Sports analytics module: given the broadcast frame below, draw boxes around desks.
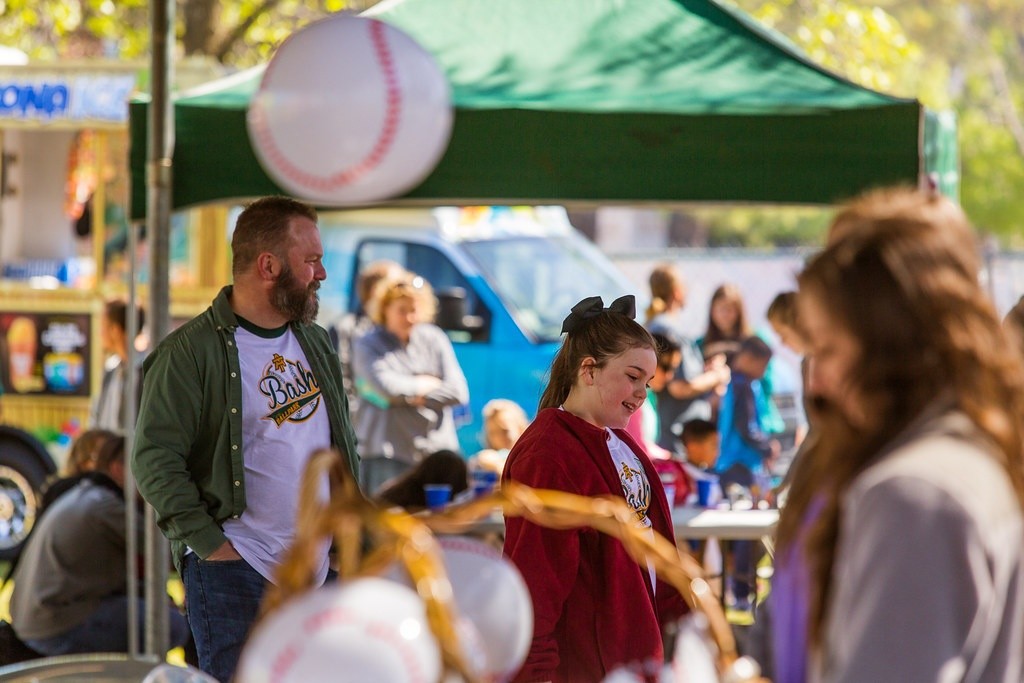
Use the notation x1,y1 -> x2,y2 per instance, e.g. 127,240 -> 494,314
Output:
670,507 -> 779,615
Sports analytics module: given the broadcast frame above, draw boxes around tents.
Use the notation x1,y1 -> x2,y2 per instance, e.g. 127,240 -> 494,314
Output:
125,0 -> 962,660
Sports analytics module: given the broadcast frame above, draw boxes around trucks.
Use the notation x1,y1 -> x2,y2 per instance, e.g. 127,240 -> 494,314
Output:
1,59 -> 650,567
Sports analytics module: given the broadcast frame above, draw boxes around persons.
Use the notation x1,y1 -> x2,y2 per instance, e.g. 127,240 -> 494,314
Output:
9,298 -> 188,656
338,256 -> 811,611
131,196 -> 359,683
499,295 -> 695,683
747,182 -> 1023,683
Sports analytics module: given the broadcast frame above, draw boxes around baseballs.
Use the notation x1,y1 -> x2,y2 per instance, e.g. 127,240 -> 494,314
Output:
245,13 -> 457,207
234,534 -> 536,683
